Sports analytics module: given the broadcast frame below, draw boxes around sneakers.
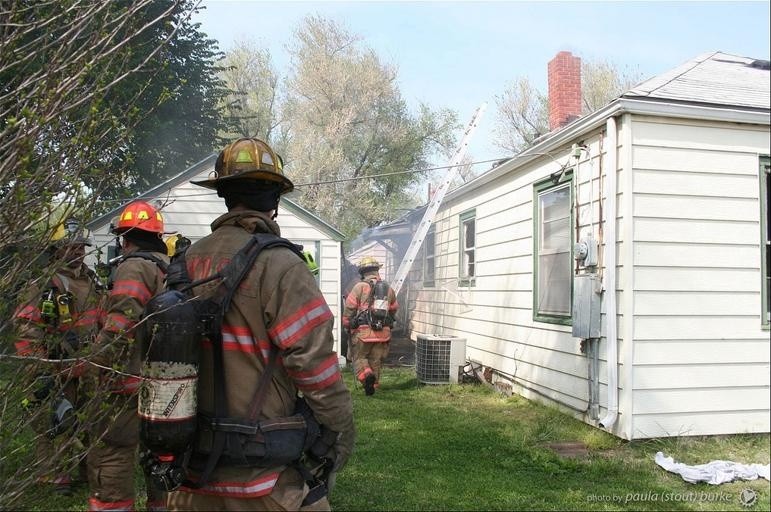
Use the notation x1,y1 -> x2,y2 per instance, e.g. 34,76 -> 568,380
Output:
364,373 -> 377,396
47,469 -> 72,500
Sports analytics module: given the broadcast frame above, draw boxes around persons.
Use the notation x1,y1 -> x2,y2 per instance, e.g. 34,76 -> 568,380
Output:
143,137 -> 356,512
343,256 -> 400,396
342,267 -> 364,367
9,198 -> 191,512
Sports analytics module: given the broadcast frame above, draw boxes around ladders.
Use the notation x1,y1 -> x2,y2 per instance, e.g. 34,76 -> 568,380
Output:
391,104 -> 486,297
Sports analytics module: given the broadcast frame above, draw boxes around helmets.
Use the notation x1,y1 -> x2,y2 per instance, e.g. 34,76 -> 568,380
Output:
112,201 -> 164,235
165,235 -> 179,258
356,257 -> 384,272
188,137 -> 295,195
48,218 -> 95,242
